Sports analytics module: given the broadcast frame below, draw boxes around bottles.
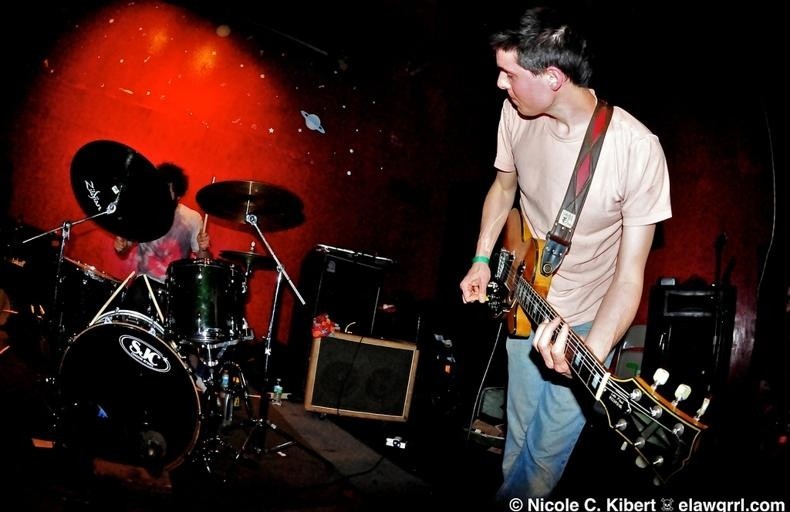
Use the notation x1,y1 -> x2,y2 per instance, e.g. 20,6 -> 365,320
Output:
221,369 -> 229,398
272,378 -> 283,404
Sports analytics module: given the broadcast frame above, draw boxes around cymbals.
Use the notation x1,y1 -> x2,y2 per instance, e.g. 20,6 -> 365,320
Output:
69,140 -> 174,243
196,179 -> 301,221
219,251 -> 279,270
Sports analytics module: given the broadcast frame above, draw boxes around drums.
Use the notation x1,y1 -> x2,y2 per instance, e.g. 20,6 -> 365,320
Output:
41,256 -> 122,330
1,223 -> 68,304
55,310 -> 204,473
162,258 -> 238,348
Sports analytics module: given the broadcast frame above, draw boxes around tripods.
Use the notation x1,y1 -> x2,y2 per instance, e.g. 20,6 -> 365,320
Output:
224,193 -> 295,469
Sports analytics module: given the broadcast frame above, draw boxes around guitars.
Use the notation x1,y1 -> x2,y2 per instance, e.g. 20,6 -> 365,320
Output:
486,207 -> 711,486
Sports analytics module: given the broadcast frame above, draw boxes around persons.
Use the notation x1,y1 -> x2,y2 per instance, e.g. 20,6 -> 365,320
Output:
112,163 -> 213,285
460,2 -> 674,512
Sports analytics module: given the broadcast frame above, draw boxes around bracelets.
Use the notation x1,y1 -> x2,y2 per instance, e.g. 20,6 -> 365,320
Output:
471,255 -> 490,265
